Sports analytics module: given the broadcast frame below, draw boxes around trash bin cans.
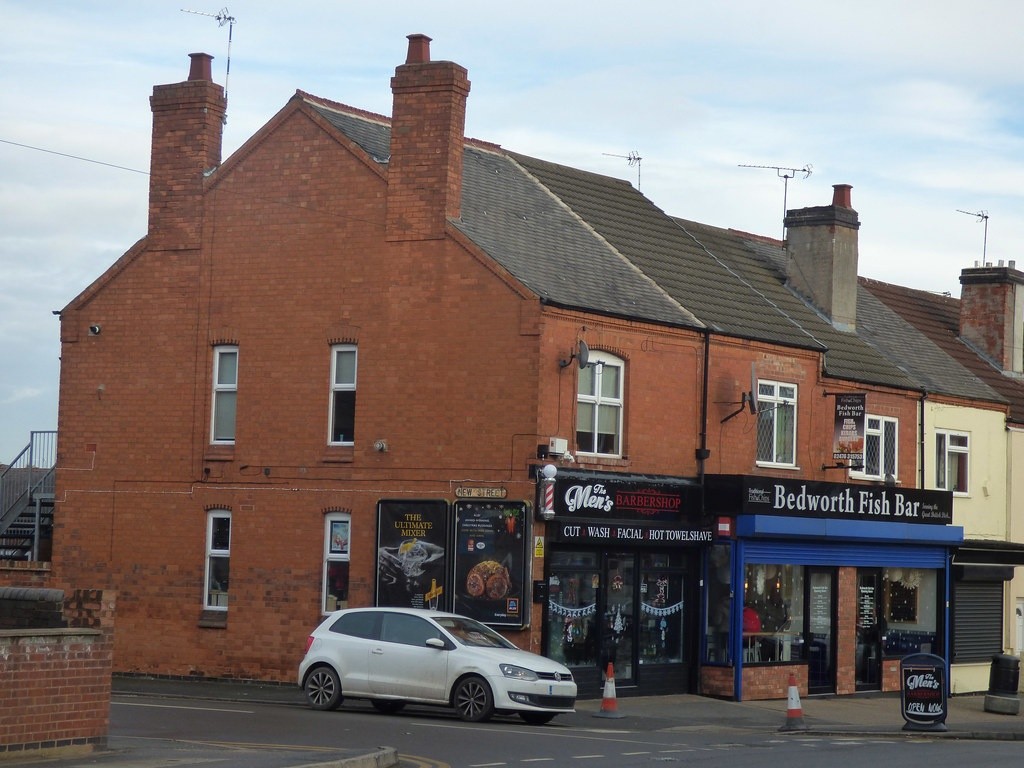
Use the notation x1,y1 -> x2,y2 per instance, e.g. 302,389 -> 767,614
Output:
988,654 -> 1021,697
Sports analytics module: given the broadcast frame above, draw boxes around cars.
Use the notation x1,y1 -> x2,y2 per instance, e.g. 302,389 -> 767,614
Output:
294,607 -> 576,728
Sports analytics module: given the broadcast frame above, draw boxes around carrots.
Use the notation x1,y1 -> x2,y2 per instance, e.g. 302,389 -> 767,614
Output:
503,508 -> 520,533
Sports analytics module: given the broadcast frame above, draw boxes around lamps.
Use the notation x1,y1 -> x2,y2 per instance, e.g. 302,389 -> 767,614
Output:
540,464 -> 557,519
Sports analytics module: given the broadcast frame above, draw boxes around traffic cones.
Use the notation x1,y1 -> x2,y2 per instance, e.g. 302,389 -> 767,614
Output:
591,663 -> 626,717
774,674 -> 818,733
